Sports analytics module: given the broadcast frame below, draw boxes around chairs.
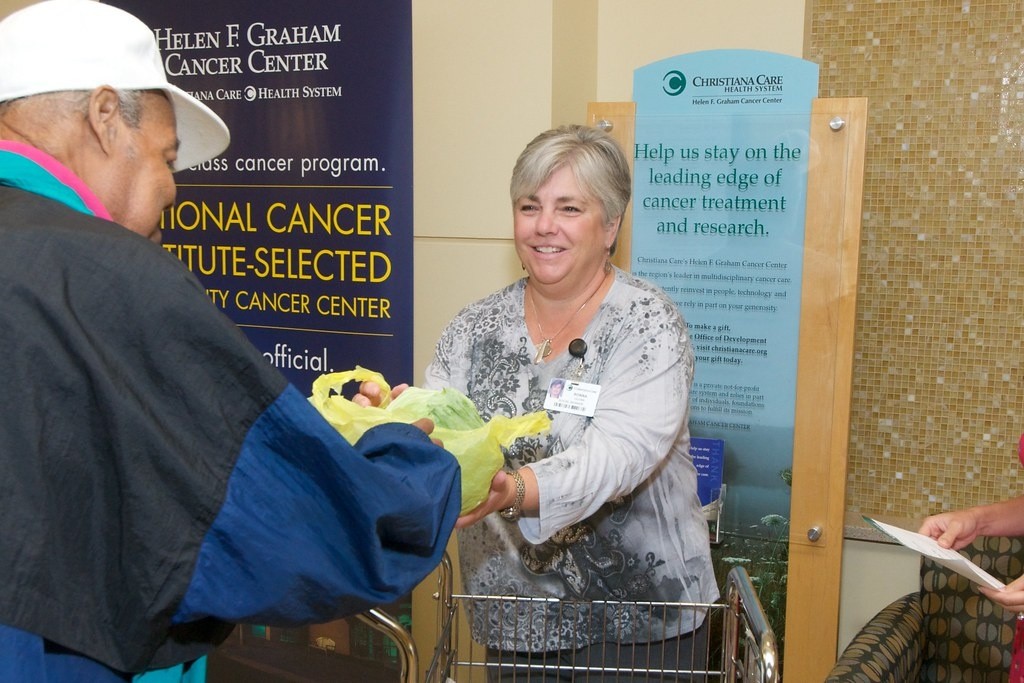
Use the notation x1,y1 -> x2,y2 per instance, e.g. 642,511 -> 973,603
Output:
823,536 -> 1024,683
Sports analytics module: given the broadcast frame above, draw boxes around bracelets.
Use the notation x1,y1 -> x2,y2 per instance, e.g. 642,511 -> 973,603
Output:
497,469 -> 526,521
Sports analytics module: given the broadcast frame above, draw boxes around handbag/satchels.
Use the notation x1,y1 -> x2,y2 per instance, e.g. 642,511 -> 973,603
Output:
305,366 -> 550,515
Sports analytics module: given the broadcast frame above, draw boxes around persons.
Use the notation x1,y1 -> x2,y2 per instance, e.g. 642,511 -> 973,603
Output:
351,125 -> 718,683
0,1 -> 464,683
917,431 -> 1023,683
549,380 -> 563,399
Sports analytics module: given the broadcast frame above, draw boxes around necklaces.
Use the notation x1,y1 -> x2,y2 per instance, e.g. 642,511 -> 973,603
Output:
528,261 -> 612,365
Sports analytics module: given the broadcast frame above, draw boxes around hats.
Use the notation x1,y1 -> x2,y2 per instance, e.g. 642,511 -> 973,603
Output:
1,0 -> 230,173
